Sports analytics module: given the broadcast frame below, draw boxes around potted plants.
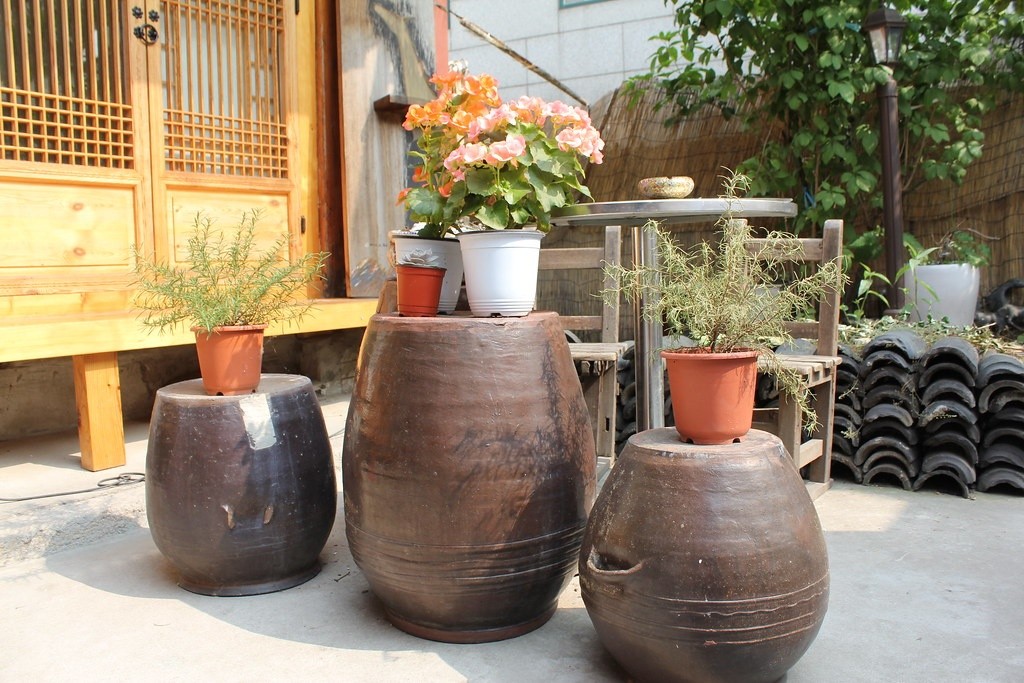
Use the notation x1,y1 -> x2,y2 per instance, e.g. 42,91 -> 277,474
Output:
904,220 -> 1002,329
122,208 -> 332,397
588,165 -> 853,444
394,248 -> 447,317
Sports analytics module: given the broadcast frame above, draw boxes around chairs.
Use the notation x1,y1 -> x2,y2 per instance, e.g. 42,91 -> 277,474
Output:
729,218 -> 844,481
537,225 -> 624,457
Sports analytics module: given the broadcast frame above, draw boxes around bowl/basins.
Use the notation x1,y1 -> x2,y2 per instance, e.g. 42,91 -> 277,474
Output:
638,175 -> 695,199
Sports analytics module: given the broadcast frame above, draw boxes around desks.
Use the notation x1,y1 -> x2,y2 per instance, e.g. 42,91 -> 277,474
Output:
541,200 -> 798,432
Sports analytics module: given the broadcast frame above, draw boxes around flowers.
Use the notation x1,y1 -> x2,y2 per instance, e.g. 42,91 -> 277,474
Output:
394,61 -> 605,238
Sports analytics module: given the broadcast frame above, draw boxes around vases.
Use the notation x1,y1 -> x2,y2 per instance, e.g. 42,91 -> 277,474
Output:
456,230 -> 544,316
392,236 -> 463,314
